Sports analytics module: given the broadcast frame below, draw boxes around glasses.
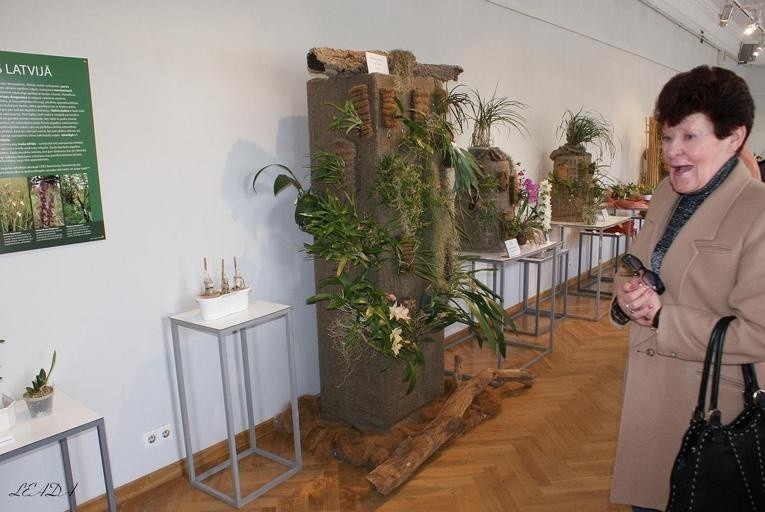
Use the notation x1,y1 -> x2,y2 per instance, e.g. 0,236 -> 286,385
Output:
618,254 -> 665,295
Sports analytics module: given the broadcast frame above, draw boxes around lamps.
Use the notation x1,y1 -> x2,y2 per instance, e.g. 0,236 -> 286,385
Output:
719,2 -> 734,28
752,33 -> 765,56
743,9 -> 761,36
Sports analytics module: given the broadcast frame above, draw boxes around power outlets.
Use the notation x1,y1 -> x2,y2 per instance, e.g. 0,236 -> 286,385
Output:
143,423 -> 174,449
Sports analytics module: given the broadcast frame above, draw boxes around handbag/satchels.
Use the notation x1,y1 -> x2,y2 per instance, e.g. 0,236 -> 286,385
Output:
663,314 -> 765,511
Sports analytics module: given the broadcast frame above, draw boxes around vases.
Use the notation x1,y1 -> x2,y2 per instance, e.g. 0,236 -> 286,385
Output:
516,233 -> 526,245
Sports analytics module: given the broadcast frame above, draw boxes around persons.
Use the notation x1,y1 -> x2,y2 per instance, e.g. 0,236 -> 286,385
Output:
609,65 -> 765,512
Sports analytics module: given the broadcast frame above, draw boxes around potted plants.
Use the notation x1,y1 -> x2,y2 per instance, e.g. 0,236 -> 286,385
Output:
549,107 -> 617,221
572,180 -> 605,224
458,81 -> 530,253
611,182 -> 656,201
0,338 -> 18,432
23,349 -> 57,417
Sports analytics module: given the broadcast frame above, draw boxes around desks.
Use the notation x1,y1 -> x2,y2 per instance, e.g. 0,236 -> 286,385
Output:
491,248 -> 568,337
445,241 -> 563,379
169,302 -> 303,509
577,215 -> 645,296
610,197 -> 652,219
526,215 -> 633,323
0,394 -> 116,511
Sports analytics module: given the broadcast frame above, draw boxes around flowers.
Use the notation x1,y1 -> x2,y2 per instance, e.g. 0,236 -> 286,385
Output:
509,179 -> 552,245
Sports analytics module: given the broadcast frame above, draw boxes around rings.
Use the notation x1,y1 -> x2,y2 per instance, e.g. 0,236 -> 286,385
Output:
625,304 -> 636,313
